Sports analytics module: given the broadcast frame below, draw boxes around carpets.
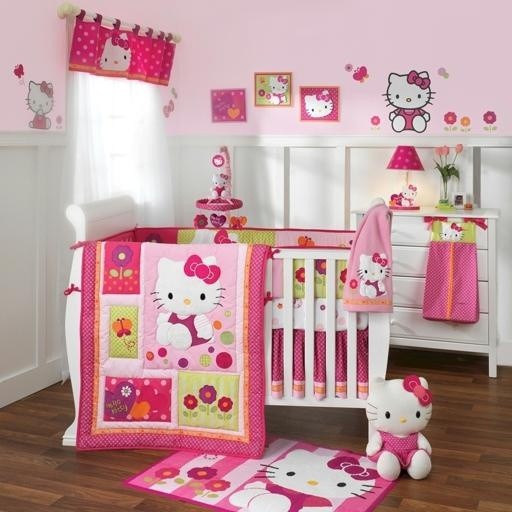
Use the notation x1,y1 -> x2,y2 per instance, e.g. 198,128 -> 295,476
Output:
122,431 -> 400,512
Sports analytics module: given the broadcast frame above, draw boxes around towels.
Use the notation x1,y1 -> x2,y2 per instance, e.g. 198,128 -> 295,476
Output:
342,204 -> 393,313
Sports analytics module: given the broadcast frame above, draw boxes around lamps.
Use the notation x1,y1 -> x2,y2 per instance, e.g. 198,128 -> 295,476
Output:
385,144 -> 426,210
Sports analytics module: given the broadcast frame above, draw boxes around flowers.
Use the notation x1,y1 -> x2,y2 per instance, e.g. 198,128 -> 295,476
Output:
432,144 -> 464,177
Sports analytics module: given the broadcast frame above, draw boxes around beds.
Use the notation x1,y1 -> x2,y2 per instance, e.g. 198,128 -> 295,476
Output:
62,194 -> 390,447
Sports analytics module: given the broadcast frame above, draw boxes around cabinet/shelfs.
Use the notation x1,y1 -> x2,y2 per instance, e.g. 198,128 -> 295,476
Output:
349,207 -> 499,378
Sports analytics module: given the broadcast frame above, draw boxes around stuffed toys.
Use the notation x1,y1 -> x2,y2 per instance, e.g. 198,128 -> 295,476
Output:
210,174 -> 230,198
365,375 -> 433,481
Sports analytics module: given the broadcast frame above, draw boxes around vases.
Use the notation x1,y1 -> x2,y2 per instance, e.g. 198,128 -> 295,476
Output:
439,176 -> 448,204
